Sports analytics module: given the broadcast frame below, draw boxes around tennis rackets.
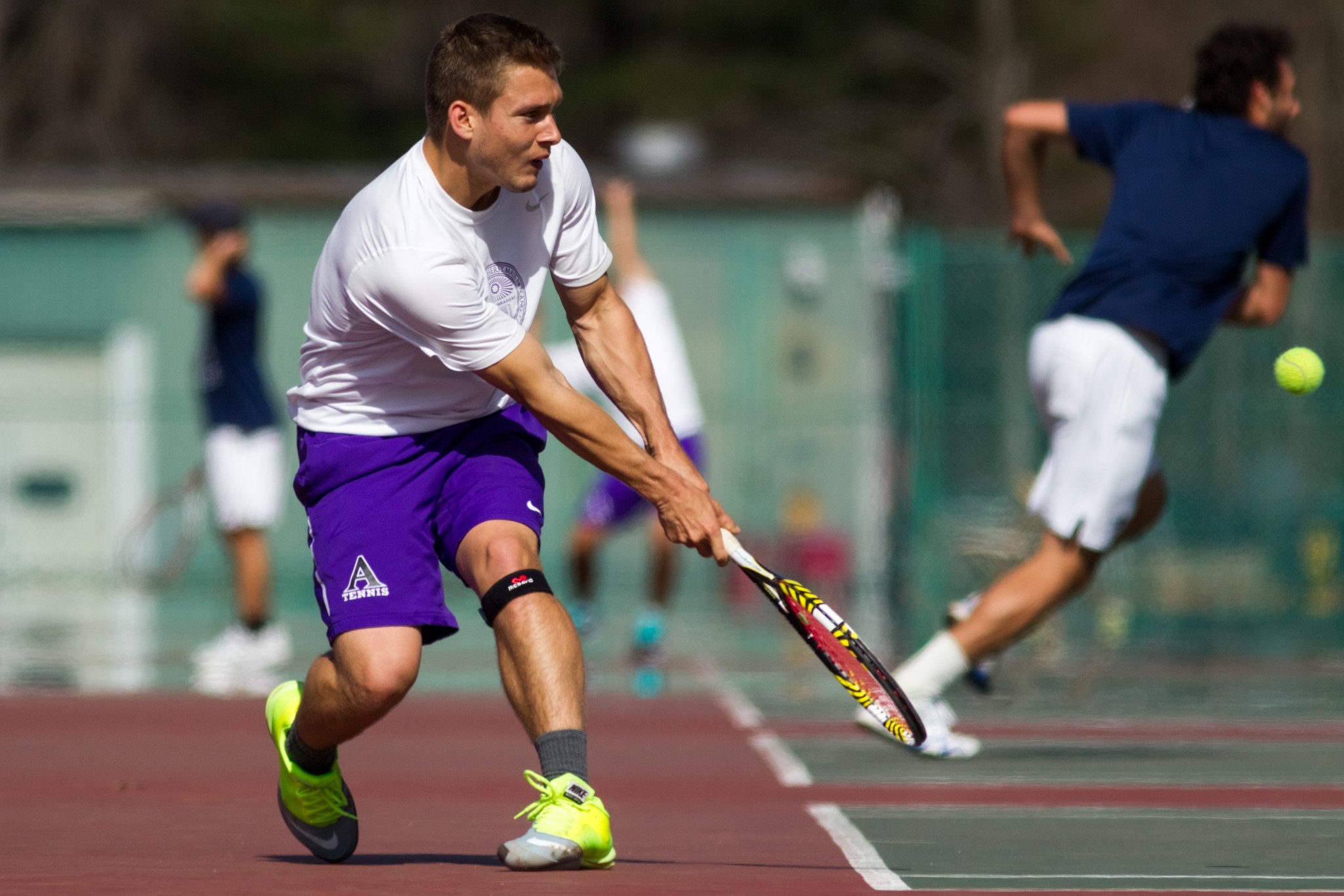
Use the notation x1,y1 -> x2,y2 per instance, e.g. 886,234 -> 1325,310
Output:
717,527 -> 928,747
118,467 -> 206,593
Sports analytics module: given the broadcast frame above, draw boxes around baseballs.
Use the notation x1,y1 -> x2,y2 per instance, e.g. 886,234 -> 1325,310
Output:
1274,347 -> 1326,394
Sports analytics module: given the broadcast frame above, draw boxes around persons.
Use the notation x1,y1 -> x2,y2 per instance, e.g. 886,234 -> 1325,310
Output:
188,201 -> 295,702
266,12 -> 739,871
534,174 -> 711,672
851,21 -> 1310,763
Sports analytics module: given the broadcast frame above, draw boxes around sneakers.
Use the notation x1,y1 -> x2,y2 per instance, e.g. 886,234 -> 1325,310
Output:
853,693 -> 981,760
946,589 -> 999,696
265,682 -> 359,860
498,772 -> 616,870
